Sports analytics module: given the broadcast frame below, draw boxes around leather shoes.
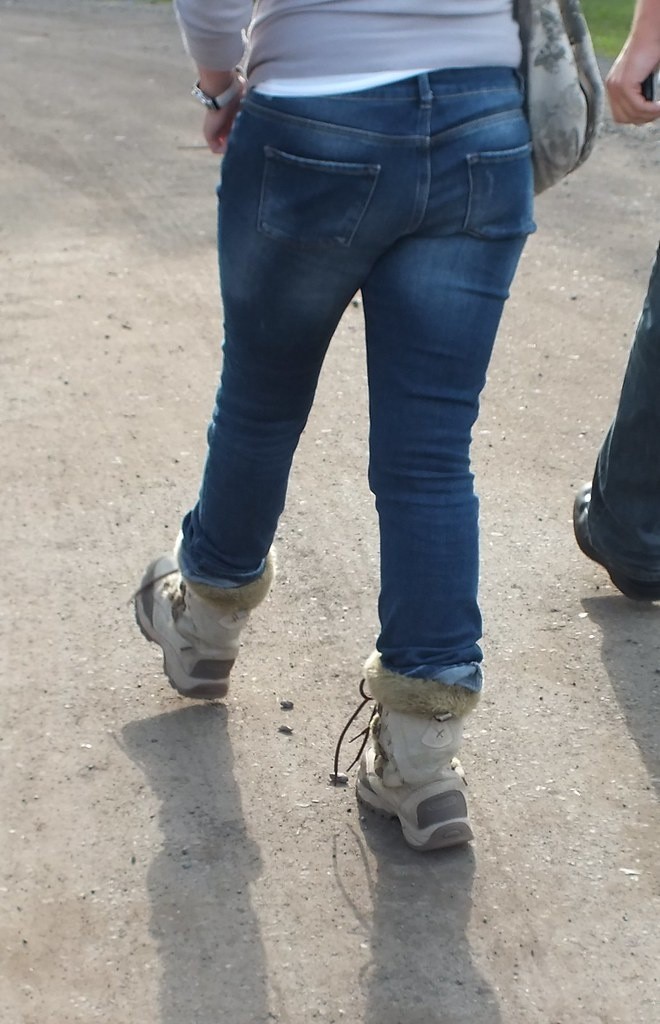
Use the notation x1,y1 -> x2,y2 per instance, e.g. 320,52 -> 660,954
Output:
572,479 -> 660,602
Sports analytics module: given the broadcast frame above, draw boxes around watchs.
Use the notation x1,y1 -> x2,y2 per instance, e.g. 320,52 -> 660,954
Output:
192,64 -> 244,110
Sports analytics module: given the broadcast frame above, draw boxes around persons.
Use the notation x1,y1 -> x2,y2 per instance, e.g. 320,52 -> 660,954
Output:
573,1 -> 659,605
126,0 -> 534,850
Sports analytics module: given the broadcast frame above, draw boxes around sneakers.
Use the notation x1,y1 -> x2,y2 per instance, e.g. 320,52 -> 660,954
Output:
354,646 -> 481,853
134,526 -> 277,700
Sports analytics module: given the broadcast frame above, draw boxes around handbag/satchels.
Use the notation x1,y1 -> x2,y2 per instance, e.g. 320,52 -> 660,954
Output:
512,0 -> 607,196
